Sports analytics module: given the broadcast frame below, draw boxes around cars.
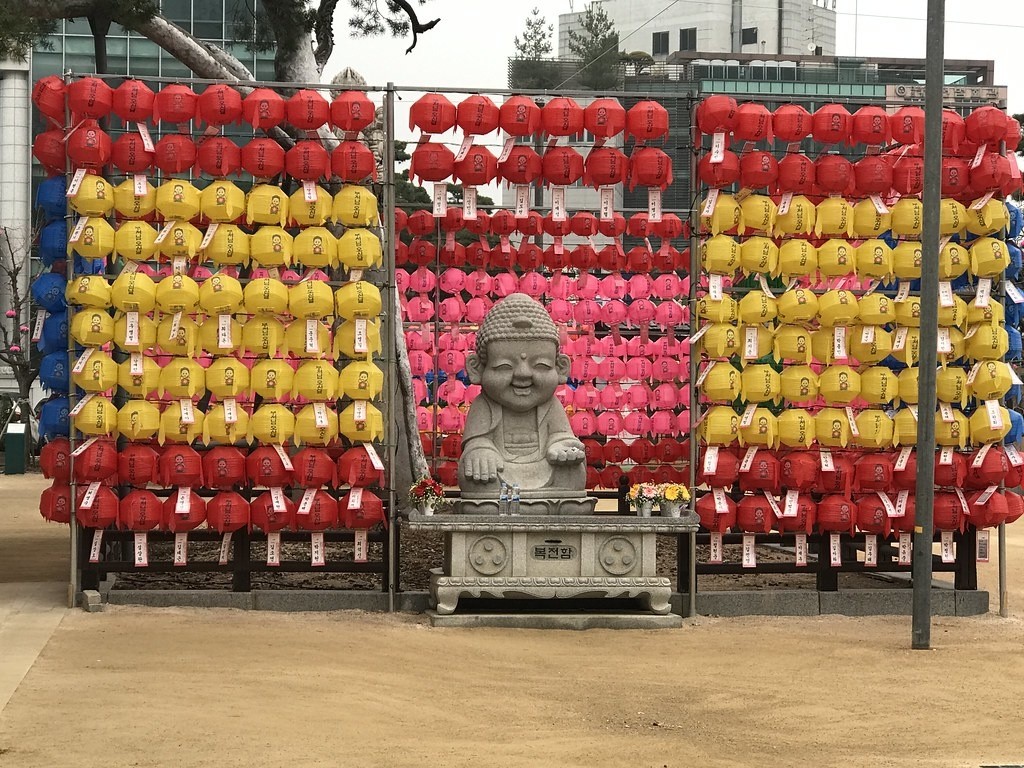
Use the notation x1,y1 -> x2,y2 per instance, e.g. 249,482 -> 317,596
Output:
0,411 -> 49,457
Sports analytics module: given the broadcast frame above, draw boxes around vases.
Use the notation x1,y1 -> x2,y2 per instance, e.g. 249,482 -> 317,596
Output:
418,501 -> 435,516
660,500 -> 681,517
636,502 -> 652,517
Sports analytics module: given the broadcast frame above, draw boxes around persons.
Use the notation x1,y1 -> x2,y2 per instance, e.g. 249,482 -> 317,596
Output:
457,292 -> 589,498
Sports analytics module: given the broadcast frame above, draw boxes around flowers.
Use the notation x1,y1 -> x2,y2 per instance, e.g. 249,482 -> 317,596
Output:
410,475 -> 444,507
624,482 -> 659,507
658,480 -> 691,509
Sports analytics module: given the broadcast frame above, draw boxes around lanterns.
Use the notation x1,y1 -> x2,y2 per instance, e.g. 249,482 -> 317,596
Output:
391,93 -> 692,486
696,96 -> 1024,536
33,75 -> 386,532
